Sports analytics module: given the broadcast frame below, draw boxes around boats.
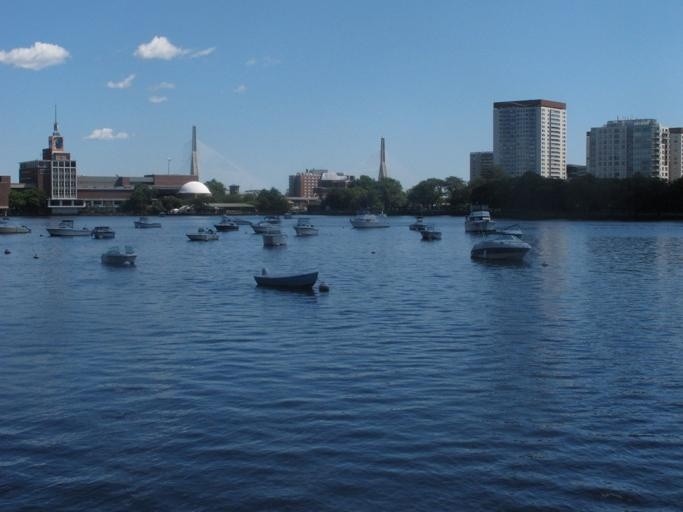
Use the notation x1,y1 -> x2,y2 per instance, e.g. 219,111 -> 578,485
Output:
459,203 -> 531,264
253,266 -> 320,290
